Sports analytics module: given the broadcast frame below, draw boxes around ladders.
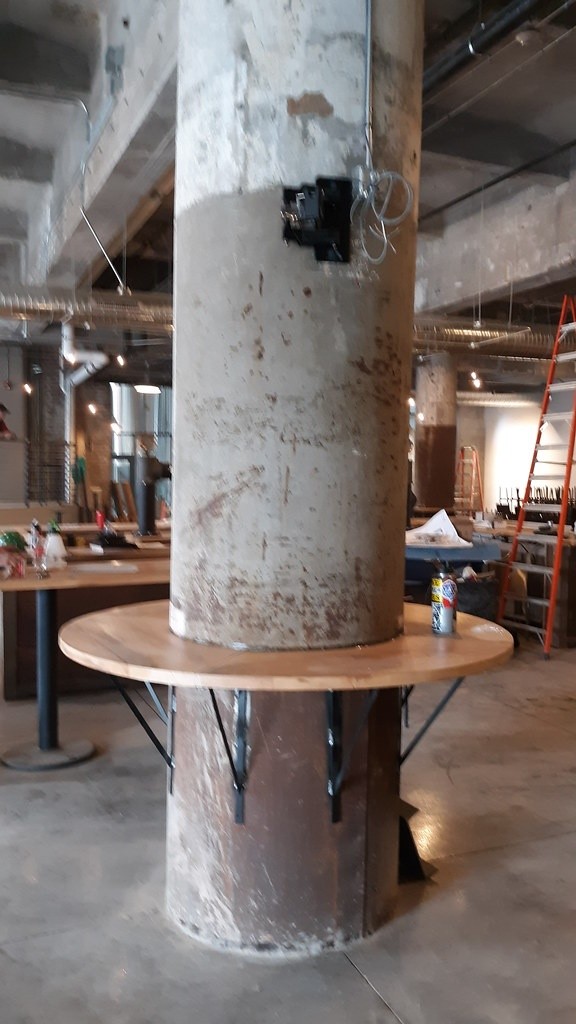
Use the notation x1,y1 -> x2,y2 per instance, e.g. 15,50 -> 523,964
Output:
454,445 -> 484,519
497,294 -> 576,661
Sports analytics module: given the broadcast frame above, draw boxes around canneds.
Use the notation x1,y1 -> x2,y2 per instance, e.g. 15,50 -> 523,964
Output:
431,572 -> 456,635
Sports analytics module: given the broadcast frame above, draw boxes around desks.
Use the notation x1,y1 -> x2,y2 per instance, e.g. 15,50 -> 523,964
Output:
0,513 -> 171,774
58,599 -> 513,825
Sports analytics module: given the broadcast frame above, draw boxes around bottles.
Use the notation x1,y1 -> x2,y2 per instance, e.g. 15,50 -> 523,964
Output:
431,567 -> 458,637
30,519 -> 50,580
